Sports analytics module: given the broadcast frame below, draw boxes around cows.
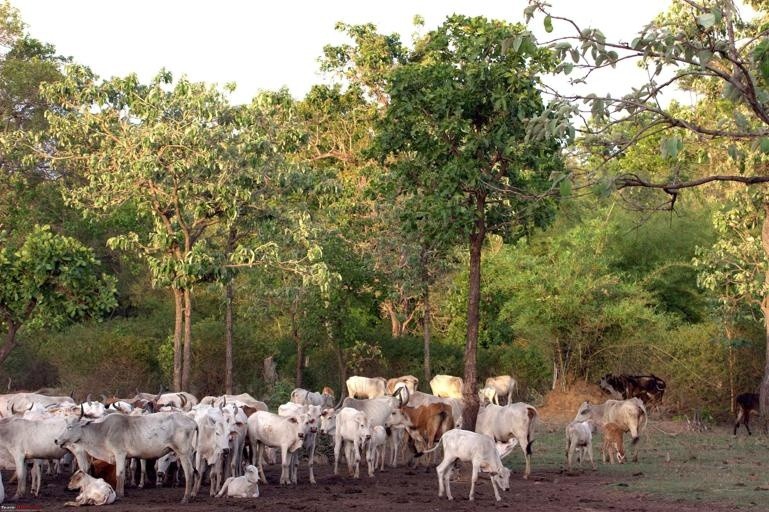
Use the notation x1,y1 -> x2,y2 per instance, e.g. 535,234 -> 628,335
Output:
0,375 -> 538,506
566,397 -> 648,473
599,373 -> 664,413
734,393 -> 760,436
